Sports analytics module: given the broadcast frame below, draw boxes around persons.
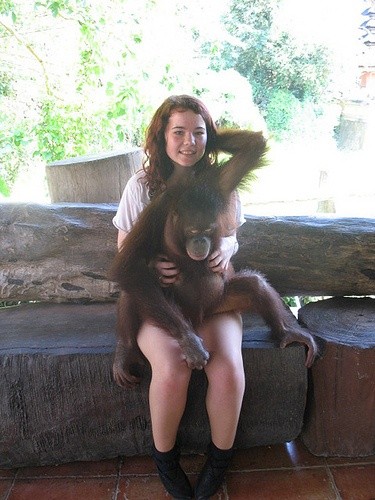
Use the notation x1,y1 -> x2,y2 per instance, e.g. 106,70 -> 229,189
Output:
113,94 -> 247,499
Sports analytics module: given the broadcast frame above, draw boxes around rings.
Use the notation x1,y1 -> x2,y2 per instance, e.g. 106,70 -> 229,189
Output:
158,274 -> 164,280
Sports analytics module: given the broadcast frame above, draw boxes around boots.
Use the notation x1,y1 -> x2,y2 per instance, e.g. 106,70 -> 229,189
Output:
152,439 -> 194,500
194,437 -> 236,500
